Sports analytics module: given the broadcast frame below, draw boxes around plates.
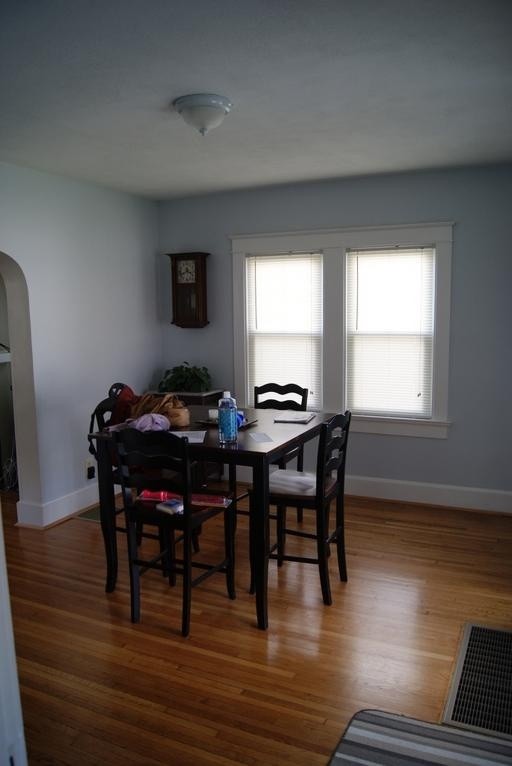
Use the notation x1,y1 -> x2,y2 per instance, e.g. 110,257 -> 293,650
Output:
194,416 -> 258,429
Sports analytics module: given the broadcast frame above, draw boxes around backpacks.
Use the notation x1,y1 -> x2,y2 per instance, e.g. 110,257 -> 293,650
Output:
93,383 -> 142,466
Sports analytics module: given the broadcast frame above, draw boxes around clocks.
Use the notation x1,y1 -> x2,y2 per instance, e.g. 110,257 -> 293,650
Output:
159,251 -> 210,329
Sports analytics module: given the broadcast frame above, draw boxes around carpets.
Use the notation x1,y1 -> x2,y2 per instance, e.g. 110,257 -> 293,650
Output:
330,705 -> 512,766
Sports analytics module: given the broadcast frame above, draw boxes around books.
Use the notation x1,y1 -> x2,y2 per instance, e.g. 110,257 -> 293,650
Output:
170,430 -> 209,443
273,408 -> 316,423
154,496 -> 184,516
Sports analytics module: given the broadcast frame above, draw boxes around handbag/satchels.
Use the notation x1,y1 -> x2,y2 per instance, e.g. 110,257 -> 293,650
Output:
125,392 -> 189,433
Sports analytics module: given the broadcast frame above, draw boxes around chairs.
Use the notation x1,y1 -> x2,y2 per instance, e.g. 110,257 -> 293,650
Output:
88,382 -> 352,638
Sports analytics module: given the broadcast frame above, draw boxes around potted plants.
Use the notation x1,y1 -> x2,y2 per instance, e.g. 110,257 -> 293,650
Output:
159,361 -> 211,393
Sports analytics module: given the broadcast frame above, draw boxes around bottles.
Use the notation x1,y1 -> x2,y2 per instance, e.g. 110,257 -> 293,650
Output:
218,391 -> 238,444
